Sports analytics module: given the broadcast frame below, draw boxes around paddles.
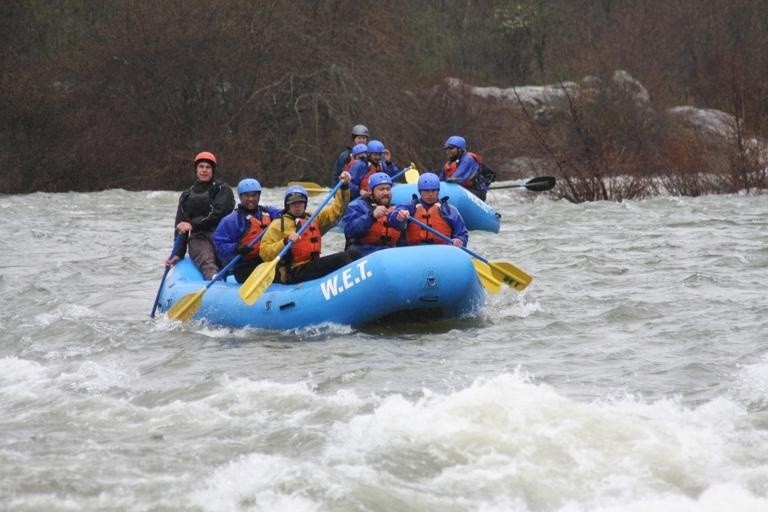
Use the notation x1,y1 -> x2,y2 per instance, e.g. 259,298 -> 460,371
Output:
239,172 -> 346,305
168,228 -> 270,323
288,182 -> 333,197
471,257 -> 505,293
478,177 -> 556,191
408,216 -> 532,291
404,168 -> 420,183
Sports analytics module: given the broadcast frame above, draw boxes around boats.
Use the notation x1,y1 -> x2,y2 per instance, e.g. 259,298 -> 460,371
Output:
157,239 -> 489,336
327,171 -> 502,235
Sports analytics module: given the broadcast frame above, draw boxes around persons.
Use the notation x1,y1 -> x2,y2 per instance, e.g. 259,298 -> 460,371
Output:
332,123 -> 488,266
165,152 -> 236,284
258,170 -> 363,284
210,178 -> 283,284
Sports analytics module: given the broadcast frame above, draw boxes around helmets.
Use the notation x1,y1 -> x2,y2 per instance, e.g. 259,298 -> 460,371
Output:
352,124 -> 369,137
418,172 -> 440,191
237,177 -> 262,194
368,172 -> 393,189
366,140 -> 385,153
284,184 -> 308,210
352,143 -> 367,157
194,151 -> 217,166
445,135 -> 466,150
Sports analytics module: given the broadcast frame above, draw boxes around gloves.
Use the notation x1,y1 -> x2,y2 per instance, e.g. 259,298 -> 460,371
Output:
237,244 -> 251,256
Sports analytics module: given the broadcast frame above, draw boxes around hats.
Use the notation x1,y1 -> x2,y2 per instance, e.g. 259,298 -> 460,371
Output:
286,192 -> 307,205
